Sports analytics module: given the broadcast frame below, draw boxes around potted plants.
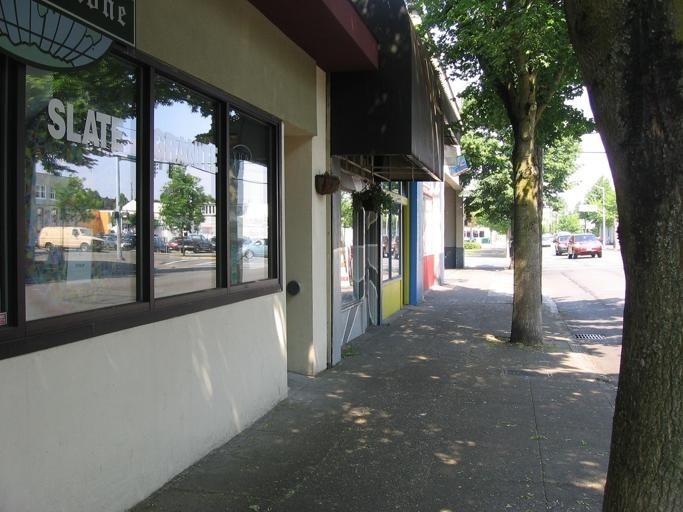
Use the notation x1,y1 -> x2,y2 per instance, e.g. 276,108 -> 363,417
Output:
351,181 -> 398,219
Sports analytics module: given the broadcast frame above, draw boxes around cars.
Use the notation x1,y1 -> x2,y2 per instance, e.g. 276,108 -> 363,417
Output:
38,226 -> 268,259
542,234 -> 603,258
